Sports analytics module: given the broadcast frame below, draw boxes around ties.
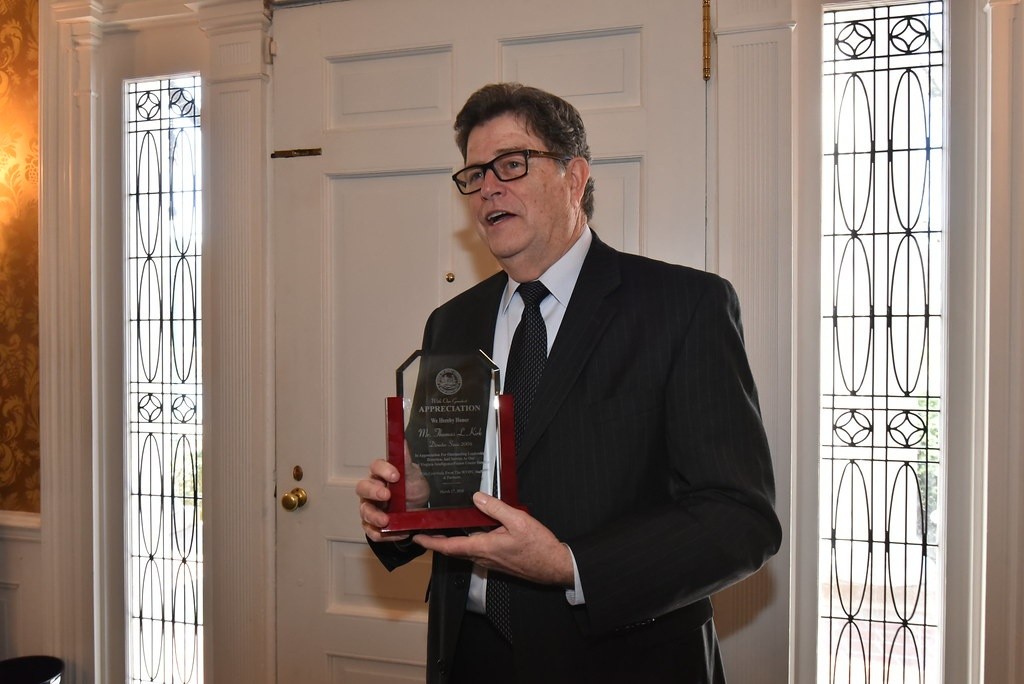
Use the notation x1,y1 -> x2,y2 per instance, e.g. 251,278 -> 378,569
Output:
485,281 -> 551,645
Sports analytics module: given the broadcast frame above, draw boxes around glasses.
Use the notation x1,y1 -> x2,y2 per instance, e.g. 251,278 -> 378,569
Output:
451,148 -> 573,195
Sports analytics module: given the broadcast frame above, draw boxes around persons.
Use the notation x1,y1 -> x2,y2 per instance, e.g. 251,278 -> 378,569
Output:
354,84 -> 784,684
